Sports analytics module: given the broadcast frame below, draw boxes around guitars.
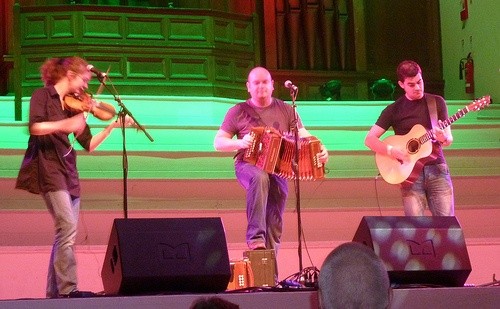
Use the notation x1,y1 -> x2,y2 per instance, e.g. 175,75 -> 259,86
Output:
375,93 -> 492,188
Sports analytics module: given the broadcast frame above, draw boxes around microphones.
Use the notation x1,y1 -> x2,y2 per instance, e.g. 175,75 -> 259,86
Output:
87,65 -> 107,77
285,80 -> 297,91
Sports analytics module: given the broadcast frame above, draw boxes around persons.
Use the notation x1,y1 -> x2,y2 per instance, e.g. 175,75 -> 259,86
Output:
14,55 -> 132,298
215,66 -> 329,290
365,60 -> 454,216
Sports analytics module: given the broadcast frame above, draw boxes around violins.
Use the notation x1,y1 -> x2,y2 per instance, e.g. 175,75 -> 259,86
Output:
62,91 -> 146,132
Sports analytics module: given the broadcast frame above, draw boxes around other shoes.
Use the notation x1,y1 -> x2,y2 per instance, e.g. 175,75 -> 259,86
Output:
249,242 -> 266,250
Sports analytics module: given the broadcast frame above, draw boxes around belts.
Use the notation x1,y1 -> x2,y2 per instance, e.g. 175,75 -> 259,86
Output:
423,158 -> 445,166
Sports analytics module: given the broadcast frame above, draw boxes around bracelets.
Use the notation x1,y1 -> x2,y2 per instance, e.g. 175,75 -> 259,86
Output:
387,145 -> 392,155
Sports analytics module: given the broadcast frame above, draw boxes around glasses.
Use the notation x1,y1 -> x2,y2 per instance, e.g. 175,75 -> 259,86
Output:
75,73 -> 88,84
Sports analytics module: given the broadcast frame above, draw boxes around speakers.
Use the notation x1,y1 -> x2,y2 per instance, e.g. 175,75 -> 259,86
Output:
101,217 -> 231,296
352,216 -> 472,287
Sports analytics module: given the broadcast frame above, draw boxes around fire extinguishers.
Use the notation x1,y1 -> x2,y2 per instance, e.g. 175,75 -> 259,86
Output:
457,51 -> 475,94
